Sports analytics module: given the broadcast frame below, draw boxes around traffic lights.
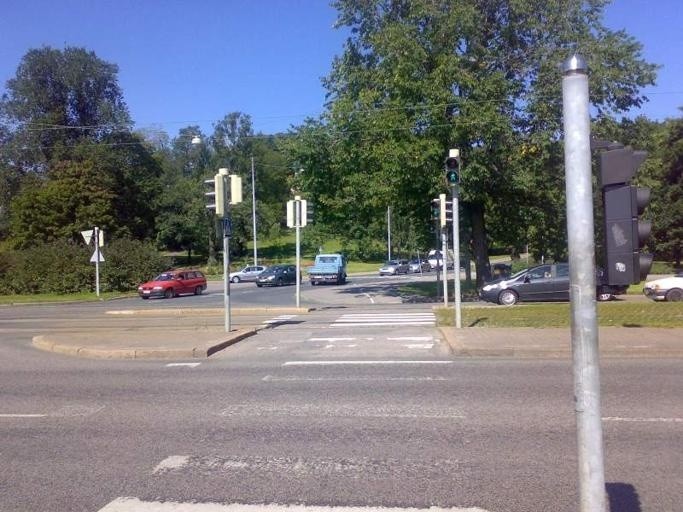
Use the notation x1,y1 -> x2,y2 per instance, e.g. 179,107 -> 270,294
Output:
203,174 -> 226,218
445,156 -> 461,189
600,144 -> 655,286
428,194 -> 454,226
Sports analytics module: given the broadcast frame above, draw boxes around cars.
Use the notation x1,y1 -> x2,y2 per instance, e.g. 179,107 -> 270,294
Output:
378,259 -> 410,276
643,269 -> 683,303
228,265 -> 268,283
138,270 -> 207,299
255,264 -> 302,288
477,261 -> 629,306
409,248 -> 456,273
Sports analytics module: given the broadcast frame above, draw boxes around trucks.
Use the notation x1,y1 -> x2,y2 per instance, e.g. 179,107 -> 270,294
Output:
306,254 -> 347,285
